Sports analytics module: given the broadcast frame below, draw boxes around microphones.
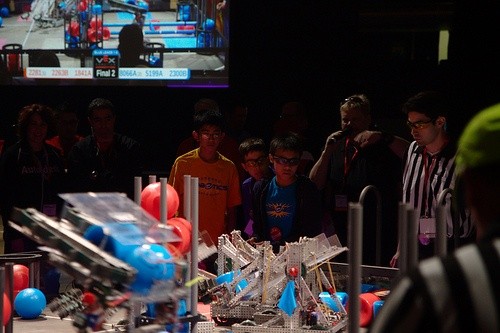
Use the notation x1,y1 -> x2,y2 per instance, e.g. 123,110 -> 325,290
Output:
327,126 -> 352,145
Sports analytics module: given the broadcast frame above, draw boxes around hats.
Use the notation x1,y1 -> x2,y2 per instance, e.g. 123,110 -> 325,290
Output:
453,103 -> 500,175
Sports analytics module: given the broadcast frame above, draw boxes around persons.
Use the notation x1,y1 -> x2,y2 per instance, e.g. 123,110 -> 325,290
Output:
367,104 -> 500,333
175,94 -> 242,166
46,105 -> 86,158
248,132 -> 336,252
220,102 -> 250,146
0,103 -> 68,253
60,98 -> 141,201
274,99 -> 328,179
309,93 -> 411,264
167,109 -> 242,247
388,92 -> 475,272
387,136 -> 412,175
237,137 -> 273,237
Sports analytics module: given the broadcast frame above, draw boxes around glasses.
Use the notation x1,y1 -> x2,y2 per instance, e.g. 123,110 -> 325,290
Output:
341,96 -> 364,106
273,156 -> 300,165
244,154 -> 269,167
407,121 -> 432,129
